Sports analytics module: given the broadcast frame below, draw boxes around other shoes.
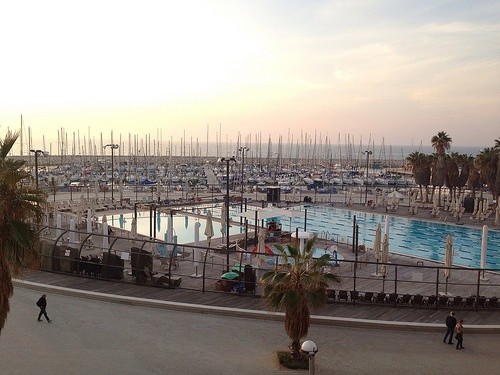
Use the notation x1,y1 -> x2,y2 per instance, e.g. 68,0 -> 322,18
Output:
461,347 -> 464,349
48,319 -> 52,322
456,347 -> 459,349
38,319 -> 42,321
443,340 -> 446,343
449,342 -> 453,344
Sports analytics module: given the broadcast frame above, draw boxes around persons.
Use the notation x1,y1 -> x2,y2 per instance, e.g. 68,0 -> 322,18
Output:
108,225 -> 114,244
455,320 -> 466,350
36,293 -> 52,323
443,311 -> 457,345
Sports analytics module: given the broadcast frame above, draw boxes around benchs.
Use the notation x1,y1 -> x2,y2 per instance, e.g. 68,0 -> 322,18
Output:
153,274 -> 181,286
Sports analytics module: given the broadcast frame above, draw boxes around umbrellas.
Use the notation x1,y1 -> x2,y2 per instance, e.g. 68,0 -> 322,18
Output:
372,215 -> 389,278
76,204 -> 82,243
220,203 -> 227,244
257,229 -> 266,269
384,187 -> 500,225
131,218 -> 137,243
444,234 -> 454,294
204,210 -> 214,253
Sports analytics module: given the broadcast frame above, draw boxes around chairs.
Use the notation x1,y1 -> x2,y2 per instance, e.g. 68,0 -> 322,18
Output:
350,290 -> 500,307
326,289 -> 336,300
338,290 -> 348,301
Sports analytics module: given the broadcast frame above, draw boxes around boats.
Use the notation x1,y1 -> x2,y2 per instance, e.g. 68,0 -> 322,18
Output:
18,112 -> 425,188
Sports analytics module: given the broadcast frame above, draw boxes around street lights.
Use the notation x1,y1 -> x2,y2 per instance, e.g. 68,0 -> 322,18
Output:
103,143 -> 119,202
217,156 -> 241,270
31,149 -> 48,236
238,146 -> 250,226
362,150 -> 373,204
